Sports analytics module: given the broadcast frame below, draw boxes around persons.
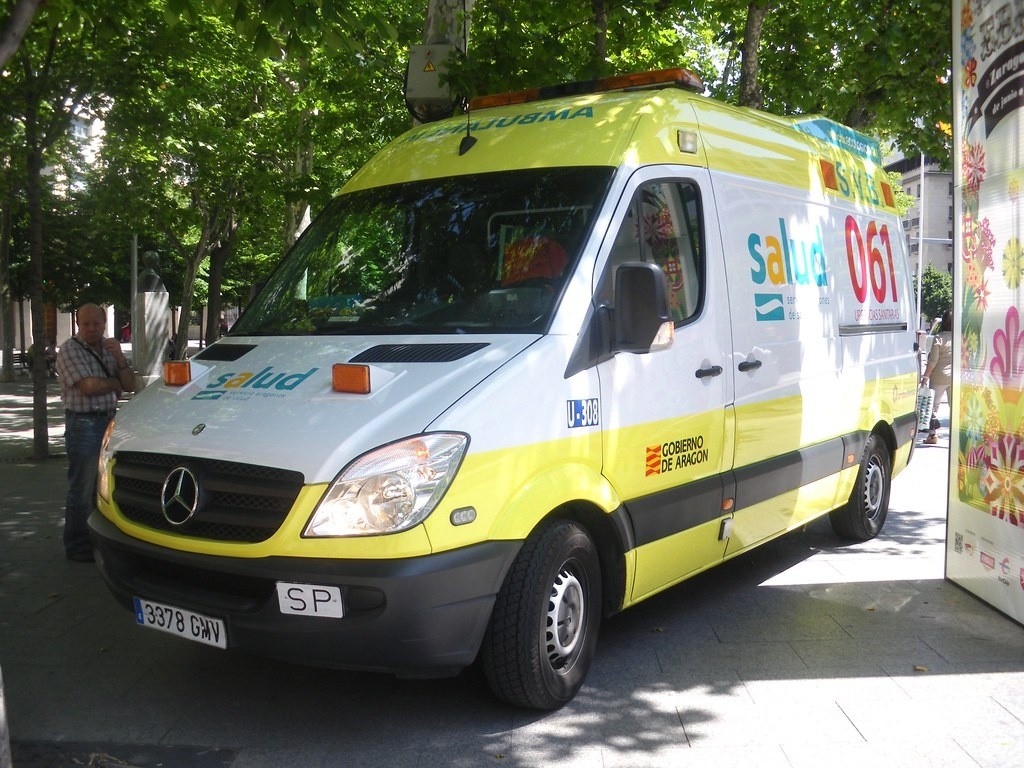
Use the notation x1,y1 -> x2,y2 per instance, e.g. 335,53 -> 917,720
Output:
118,309 -> 131,342
920,312 -> 953,444
53,303 -> 135,562
500,239 -> 653,351
219,319 -> 229,337
169,334 -> 177,359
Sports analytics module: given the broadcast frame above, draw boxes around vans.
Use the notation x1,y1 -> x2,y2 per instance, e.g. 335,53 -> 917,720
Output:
85,72 -> 926,715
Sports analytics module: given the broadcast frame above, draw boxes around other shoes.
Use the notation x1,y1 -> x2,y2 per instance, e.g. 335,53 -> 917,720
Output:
65,549 -> 94,563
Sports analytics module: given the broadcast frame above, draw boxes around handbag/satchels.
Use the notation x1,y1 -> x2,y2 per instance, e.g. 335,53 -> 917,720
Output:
917,381 -> 935,430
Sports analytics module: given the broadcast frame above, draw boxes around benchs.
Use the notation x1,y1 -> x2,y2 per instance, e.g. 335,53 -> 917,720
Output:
13,353 -> 28,363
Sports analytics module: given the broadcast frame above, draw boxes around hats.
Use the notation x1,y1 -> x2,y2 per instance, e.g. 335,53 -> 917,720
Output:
500,238 -> 568,286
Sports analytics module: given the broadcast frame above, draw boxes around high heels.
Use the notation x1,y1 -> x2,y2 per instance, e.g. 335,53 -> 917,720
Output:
923,434 -> 938,444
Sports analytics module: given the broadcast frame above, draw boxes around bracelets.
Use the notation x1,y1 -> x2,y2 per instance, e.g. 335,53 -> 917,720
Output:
922,375 -> 928,378
118,357 -> 132,369
